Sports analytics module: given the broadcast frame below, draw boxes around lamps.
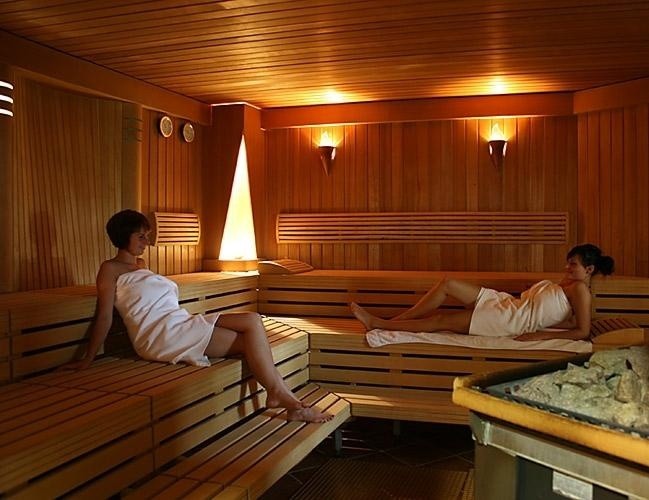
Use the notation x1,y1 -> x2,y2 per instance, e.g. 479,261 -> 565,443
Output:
314,129 -> 336,176
485,121 -> 509,168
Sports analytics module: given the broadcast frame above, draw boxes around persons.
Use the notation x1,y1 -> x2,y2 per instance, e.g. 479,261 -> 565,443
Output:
350,242 -> 615,342
58,208 -> 335,423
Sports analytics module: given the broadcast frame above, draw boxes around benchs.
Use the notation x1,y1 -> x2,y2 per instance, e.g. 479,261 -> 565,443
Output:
0,263 -> 649,500
